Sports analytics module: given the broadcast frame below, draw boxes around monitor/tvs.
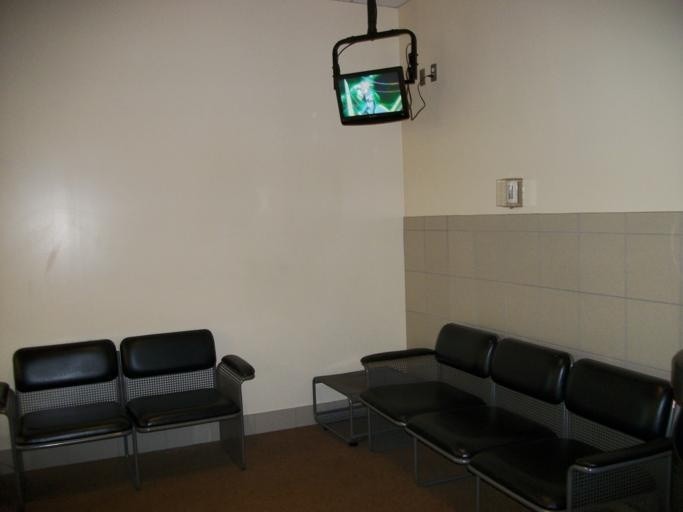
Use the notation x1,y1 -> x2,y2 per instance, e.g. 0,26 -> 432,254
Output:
336,66 -> 410,125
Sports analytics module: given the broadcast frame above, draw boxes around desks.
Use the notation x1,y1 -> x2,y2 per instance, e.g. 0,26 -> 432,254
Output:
312,370 -> 367,447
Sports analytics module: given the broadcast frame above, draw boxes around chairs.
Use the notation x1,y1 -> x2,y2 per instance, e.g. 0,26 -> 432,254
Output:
3,340 -> 132,489
359,324 -> 501,488
122,329 -> 254,489
406,342 -> 573,510
466,356 -> 679,512
